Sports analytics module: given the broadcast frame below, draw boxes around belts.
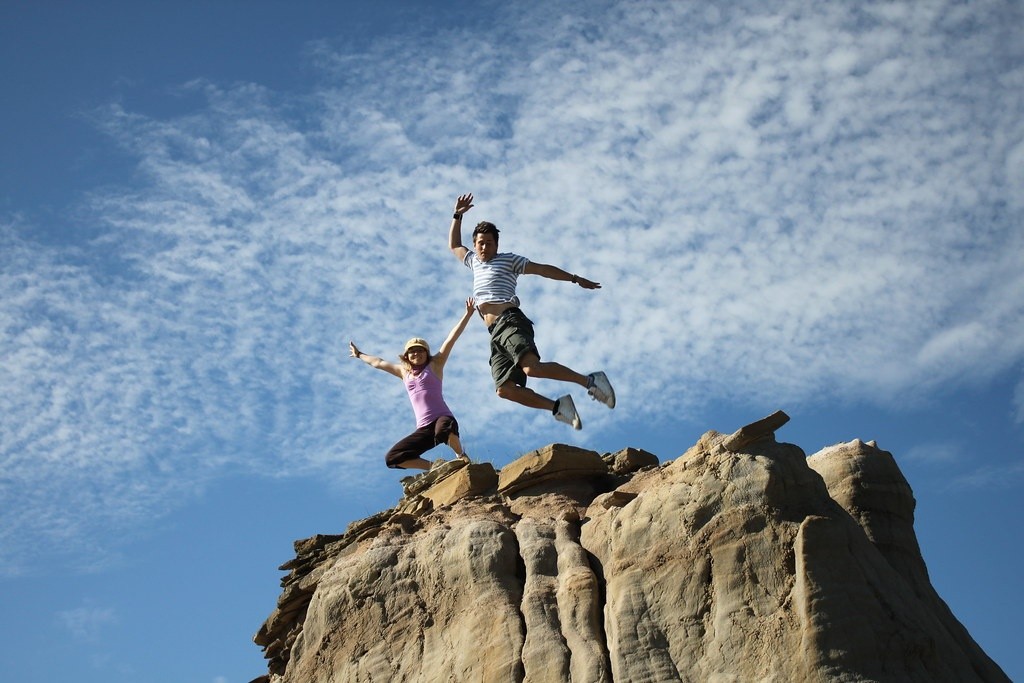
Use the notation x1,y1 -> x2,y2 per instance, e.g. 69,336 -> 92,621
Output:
488,307 -> 520,334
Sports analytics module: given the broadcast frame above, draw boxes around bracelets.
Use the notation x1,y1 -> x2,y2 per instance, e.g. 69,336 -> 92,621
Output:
453,213 -> 462,220
357,352 -> 361,358
572,274 -> 577,283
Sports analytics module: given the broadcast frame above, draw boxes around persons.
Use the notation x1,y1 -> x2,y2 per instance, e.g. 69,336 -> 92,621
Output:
348,297 -> 476,471
448,192 -> 616,430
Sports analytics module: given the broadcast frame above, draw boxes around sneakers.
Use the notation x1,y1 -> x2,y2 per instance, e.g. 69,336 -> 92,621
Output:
588,371 -> 615,409
554,394 -> 582,430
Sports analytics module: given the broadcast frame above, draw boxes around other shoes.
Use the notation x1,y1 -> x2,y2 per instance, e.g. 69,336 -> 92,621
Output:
457,447 -> 471,464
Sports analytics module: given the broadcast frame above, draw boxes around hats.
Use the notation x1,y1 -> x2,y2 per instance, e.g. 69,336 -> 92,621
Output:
404,338 -> 429,353
